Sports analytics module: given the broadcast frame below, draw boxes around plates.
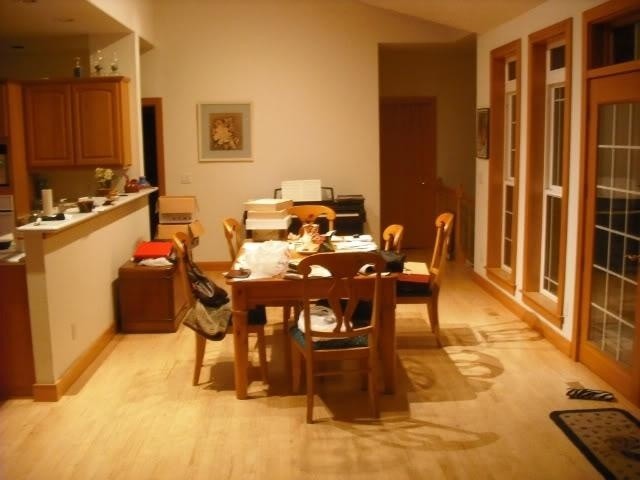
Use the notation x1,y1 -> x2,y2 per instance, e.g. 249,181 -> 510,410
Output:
288,236 -> 335,254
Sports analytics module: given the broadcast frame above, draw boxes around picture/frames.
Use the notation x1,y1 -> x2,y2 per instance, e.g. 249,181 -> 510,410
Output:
196,103 -> 254,162
475,108 -> 489,158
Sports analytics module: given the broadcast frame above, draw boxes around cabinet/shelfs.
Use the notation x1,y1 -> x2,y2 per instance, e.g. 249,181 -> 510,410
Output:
21,75 -> 132,168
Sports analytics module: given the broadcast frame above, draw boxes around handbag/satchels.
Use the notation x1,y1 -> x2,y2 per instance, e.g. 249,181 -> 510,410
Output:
182,251 -> 230,306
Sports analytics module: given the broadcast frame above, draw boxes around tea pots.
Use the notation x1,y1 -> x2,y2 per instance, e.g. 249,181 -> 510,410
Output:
124,179 -> 141,194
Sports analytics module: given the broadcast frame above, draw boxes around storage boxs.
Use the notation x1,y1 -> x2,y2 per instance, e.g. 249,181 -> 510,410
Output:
155,196 -> 204,248
118,242 -> 194,333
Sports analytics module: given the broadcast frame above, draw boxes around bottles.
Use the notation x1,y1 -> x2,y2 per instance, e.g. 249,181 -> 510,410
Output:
138,176 -> 148,187
41,189 -> 54,216
73,57 -> 82,78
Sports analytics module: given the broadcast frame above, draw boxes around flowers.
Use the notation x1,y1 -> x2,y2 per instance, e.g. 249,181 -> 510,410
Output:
93,167 -> 115,189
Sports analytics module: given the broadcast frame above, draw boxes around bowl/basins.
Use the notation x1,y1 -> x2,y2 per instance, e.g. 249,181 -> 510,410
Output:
0,241 -> 11,250
77,201 -> 93,215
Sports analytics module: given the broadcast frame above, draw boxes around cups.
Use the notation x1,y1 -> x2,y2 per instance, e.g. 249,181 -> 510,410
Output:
93,49 -> 105,76
110,51 -> 121,77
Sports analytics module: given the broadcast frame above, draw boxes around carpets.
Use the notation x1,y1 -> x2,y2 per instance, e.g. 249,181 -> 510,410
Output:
549,408 -> 640,480
